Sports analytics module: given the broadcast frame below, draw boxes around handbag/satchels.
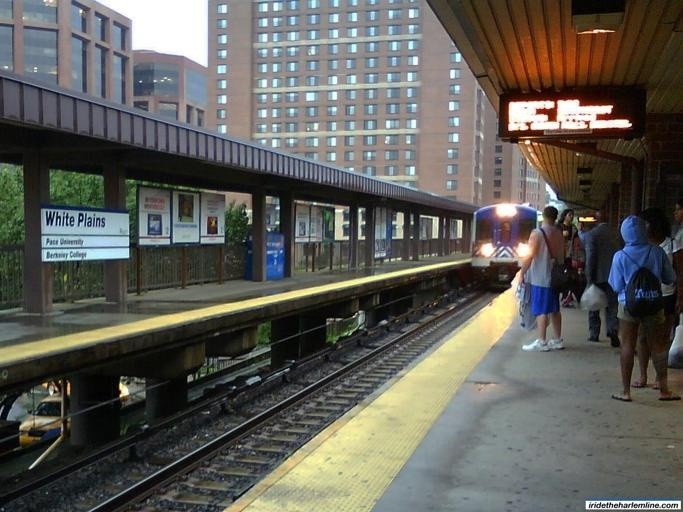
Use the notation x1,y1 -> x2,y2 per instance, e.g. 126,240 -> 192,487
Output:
550,262 -> 580,293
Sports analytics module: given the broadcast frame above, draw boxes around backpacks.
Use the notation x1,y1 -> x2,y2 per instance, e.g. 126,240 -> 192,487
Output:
620,245 -> 663,318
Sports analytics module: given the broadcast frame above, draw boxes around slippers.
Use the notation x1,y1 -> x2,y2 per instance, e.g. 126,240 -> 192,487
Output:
631,380 -> 648,388
610,389 -> 632,402
652,383 -> 660,390
658,392 -> 682,401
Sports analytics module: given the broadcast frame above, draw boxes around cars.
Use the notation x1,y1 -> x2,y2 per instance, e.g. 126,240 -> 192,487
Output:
43,380 -> 128,404
18,392 -> 70,447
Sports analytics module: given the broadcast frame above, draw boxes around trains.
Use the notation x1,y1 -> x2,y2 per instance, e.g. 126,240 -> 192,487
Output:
472,201 -> 544,287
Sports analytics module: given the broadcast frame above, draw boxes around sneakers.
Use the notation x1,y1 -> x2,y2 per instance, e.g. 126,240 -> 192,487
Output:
546,337 -> 566,351
586,336 -> 602,342
522,337 -> 549,352
609,331 -> 621,347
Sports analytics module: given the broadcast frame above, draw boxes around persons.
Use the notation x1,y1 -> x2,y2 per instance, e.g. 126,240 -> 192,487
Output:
517,197 -> 683,403
